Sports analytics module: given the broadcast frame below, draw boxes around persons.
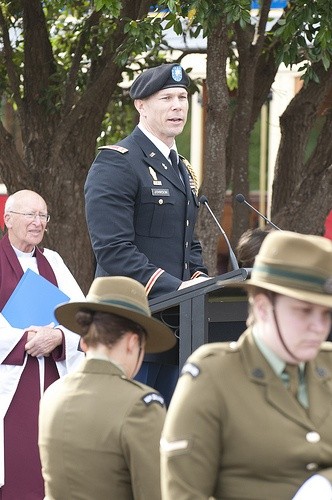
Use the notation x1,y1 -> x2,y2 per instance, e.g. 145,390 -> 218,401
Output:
0,191 -> 86,500
83,65 -> 212,408
159,229 -> 331,500
39,276 -> 165,500
236,229 -> 267,266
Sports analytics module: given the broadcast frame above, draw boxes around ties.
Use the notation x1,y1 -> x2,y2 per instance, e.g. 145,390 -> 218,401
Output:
169,150 -> 179,177
285,366 -> 299,394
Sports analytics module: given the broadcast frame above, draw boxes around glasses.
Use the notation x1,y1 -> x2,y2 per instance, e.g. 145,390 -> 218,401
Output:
10,211 -> 50,222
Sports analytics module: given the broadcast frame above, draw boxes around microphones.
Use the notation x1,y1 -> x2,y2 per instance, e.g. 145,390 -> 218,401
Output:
235,193 -> 282,231
199,195 -> 239,271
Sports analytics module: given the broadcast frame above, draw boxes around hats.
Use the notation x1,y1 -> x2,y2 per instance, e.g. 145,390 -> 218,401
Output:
218,230 -> 332,307
130,63 -> 190,99
54,276 -> 176,354
